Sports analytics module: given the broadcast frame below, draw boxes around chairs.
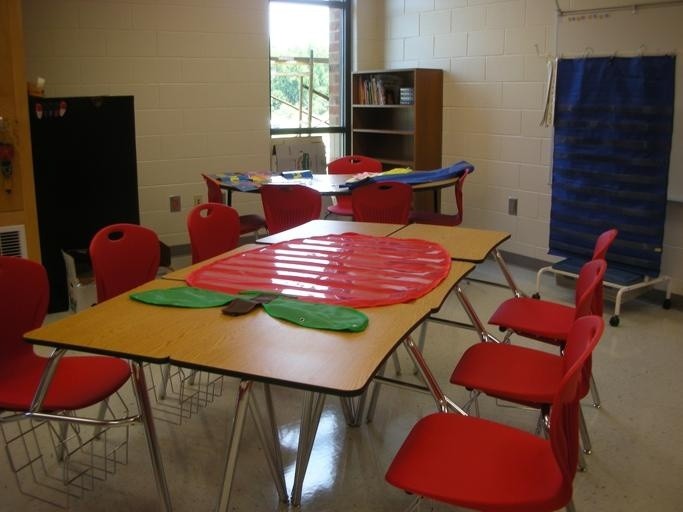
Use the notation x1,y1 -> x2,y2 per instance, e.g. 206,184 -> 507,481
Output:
59,248 -> 98,314
88,224 -> 160,304
201,172 -> 268,242
187,203 -> 247,266
0,255 -> 131,425
448,258 -> 607,454
186,202 -> 241,386
259,184 -> 322,236
408,168 -> 469,225
350,181 -> 411,225
486,228 -> 618,409
323,156 -> 381,221
384,315 -> 604,511
89,225 -> 172,440
155,241 -> 176,279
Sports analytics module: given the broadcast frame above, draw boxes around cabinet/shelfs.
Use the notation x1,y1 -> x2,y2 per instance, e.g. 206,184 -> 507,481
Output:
350,68 -> 443,213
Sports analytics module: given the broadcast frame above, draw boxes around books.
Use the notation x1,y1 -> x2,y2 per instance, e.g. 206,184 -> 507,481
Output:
357,74 -> 413,107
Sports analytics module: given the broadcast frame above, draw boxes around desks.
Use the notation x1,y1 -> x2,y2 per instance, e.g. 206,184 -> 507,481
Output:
212,170 -> 460,196
23,218 -> 526,512
